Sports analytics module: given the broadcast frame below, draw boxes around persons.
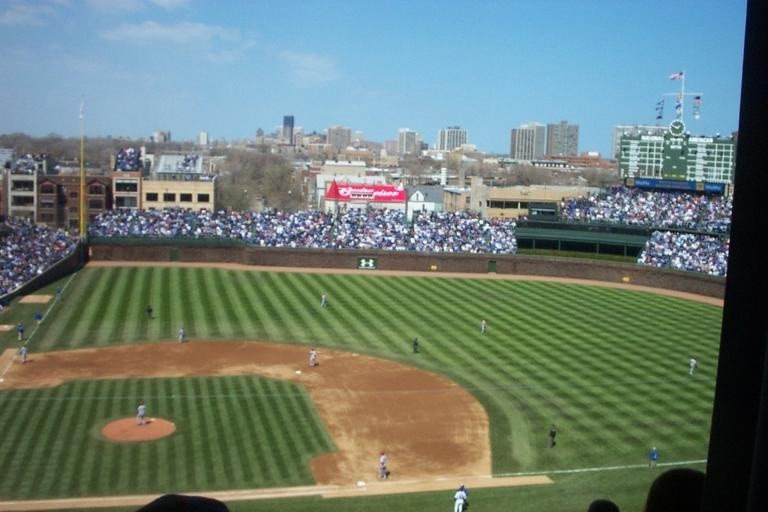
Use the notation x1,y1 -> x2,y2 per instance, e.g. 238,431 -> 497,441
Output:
19,345 -> 30,363
411,336 -> 420,357
35,310 -> 42,328
459,483 -> 468,509
13,152 -> 44,173
561,187 -> 734,233
647,446 -> 660,467
587,499 -> 620,511
690,355 -> 696,374
307,347 -> 316,366
319,293 -> 328,306
378,449 -> 388,480
480,317 -> 486,335
114,147 -> 140,170
177,326 -> 185,344
135,400 -> 147,424
549,424 -> 556,448
454,487 -> 468,512
144,304 -> 154,318
14,322 -> 26,339
1,215 -> 76,299
86,204 -> 518,256
638,233 -> 728,280
645,468 -> 706,511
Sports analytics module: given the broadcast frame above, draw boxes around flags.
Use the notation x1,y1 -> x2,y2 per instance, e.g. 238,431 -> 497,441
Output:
694,96 -> 701,114
675,96 -> 683,115
669,71 -> 684,80
655,100 -> 664,118
